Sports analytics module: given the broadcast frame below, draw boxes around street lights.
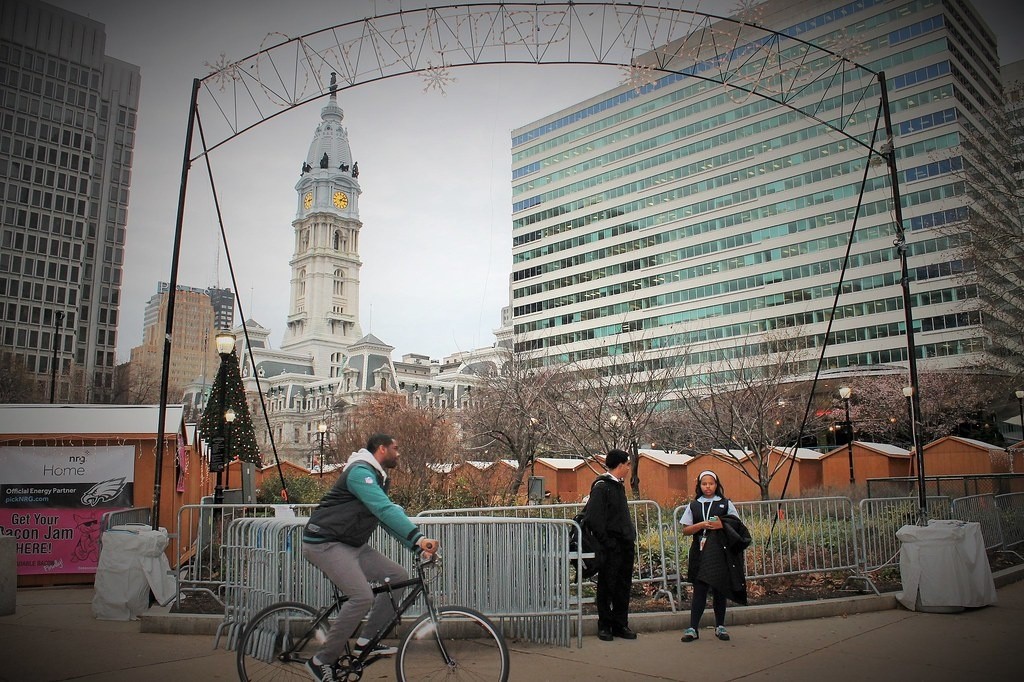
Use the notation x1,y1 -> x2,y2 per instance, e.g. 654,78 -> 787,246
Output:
318,419 -> 327,478
528,413 -> 539,476
838,380 -> 856,491
213,320 -> 237,520
901,384 -> 915,446
1015,386 -> 1024,441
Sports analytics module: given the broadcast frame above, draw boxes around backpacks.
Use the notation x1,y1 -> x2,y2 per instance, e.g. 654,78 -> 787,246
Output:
569,480 -> 605,583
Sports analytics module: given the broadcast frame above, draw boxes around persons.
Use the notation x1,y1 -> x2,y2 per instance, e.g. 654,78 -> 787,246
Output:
586,449 -> 637,642
679,470 -> 739,642
302,434 -> 439,682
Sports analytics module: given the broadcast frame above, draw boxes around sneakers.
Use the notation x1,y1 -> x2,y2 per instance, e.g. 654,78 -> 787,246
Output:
304,656 -> 337,682
715,625 -> 730,639
612,624 -> 636,639
681,627 -> 699,641
597,627 -> 613,641
354,641 -> 398,656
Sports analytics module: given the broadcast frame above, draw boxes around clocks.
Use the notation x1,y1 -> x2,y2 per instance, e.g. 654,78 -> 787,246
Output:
333,191 -> 349,209
303,191 -> 313,209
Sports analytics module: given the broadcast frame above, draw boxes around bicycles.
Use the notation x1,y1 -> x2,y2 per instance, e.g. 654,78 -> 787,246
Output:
236,542 -> 510,682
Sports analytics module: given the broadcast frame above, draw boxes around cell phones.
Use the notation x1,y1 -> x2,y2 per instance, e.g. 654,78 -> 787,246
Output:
710,517 -> 717,521
